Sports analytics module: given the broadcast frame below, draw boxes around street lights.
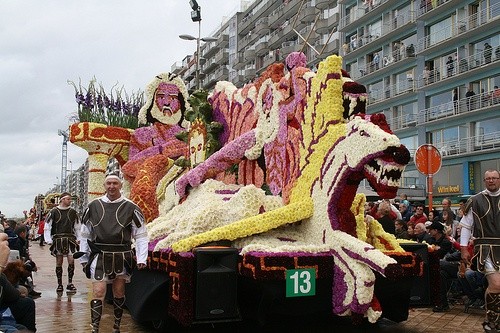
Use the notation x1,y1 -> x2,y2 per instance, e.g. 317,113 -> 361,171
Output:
178,0 -> 218,91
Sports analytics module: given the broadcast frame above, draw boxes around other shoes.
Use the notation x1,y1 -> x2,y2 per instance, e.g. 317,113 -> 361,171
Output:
40,244 -> 45,246
30,290 -> 41,296
433,305 -> 449,312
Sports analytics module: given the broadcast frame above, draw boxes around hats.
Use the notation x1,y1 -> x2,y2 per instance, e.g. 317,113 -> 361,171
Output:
426,222 -> 444,232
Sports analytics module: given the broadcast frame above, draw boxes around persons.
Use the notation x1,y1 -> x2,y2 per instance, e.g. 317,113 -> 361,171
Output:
0,212 -> 41,333
393,43 -> 414,62
373,53 -> 380,70
43,192 -> 81,290
420,0 -> 446,14
38,218 -> 45,246
423,66 -> 430,85
459,169 -> 500,333
342,42 -> 349,54
363,0 -> 382,13
446,56 -> 454,77
484,43 -> 492,64
453,89 -> 458,115
466,89 -> 476,111
365,193 -> 488,313
79,174 -> 148,333
481,86 -> 500,108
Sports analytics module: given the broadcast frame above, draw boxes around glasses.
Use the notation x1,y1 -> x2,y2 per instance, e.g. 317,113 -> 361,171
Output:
484,177 -> 500,182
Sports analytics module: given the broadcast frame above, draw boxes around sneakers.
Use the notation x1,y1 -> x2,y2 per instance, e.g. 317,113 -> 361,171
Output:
66,284 -> 77,292
57,285 -> 62,291
